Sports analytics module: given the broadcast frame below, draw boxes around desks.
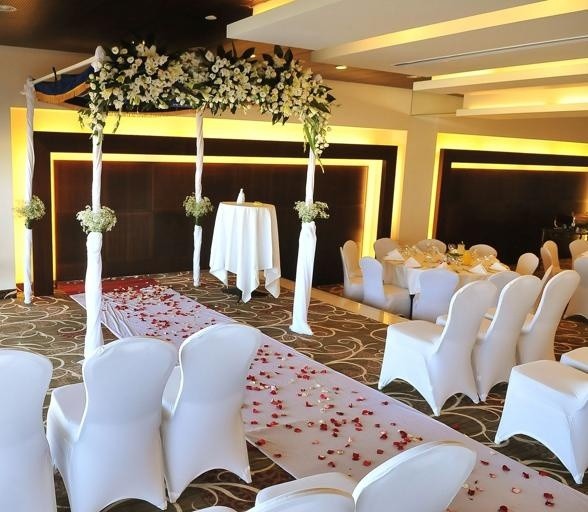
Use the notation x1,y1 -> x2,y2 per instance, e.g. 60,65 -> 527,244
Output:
208,202 -> 281,304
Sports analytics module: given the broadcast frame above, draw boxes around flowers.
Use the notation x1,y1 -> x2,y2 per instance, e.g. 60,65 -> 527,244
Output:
76,39 -> 336,174
12,192 -> 46,229
182,191 -> 214,226
76,205 -> 117,234
293,200 -> 329,223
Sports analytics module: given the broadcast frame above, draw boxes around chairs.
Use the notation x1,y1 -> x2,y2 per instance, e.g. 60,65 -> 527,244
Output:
343,240 -> 362,279
158,321 -> 260,503
359,257 -> 411,318
254,440 -> 478,511
436,274 -> 543,403
377,278 -> 498,417
538,265 -> 553,282
515,252 -> 539,277
190,488 -> 355,512
540,247 -> 555,276
563,256 -> 588,320
46,334 -> 178,512
485,269 -> 581,364
559,345 -> 588,374
469,244 -> 497,258
340,247 -> 363,301
412,268 -> 459,323
0,347 -> 58,512
373,238 -> 400,259
488,271 -> 520,294
569,239 -> 587,270
543,240 -> 562,273
495,360 -> 587,484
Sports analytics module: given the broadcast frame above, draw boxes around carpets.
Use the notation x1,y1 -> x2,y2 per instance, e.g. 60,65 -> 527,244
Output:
70,285 -> 588,511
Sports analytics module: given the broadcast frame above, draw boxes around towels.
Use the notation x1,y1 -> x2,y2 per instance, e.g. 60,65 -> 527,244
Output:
403,257 -> 422,268
385,252 -> 404,261
489,262 -> 507,272
389,249 -> 400,255
237,189 -> 245,204
437,262 -> 452,270
468,263 -> 490,276
